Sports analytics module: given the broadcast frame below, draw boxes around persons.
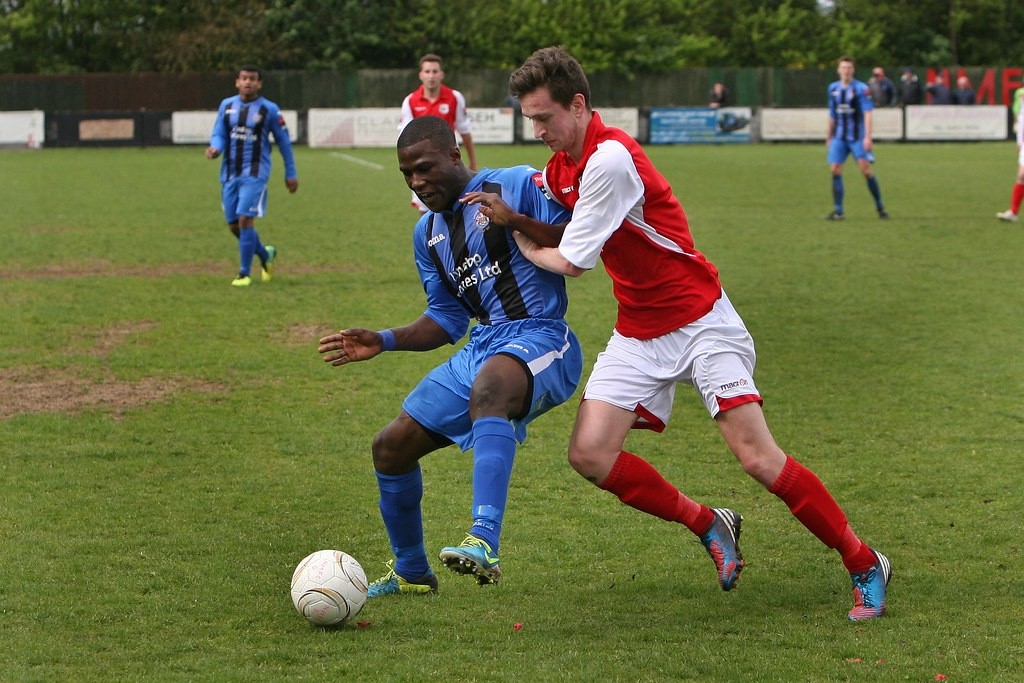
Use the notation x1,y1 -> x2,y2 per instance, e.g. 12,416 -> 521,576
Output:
819,54 -> 892,224
1012,86 -> 1024,135
865,66 -> 898,108
950,76 -> 975,105
317,115 -> 584,596
201,66 -> 303,291
993,92 -> 1024,221
403,49 -> 479,224
498,46 -> 897,625
898,66 -> 923,106
708,79 -> 735,111
925,76 -> 953,104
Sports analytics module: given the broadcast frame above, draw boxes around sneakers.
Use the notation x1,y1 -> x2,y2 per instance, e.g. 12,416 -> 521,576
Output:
262,246 -> 277,281
877,210 -> 888,218
848,548 -> 893,622
439,539 -> 501,587
700,508 -> 745,591
367,571 -> 438,596
825,211 -> 847,220
997,209 -> 1018,222
231,274 -> 252,286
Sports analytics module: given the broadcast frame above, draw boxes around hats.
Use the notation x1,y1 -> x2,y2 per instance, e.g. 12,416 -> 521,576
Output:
903,66 -> 913,70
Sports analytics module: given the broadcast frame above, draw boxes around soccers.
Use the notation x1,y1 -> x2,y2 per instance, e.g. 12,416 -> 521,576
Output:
290,549 -> 372,627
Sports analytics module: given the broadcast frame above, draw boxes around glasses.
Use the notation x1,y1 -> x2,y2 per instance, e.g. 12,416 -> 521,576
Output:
873,73 -> 879,76
